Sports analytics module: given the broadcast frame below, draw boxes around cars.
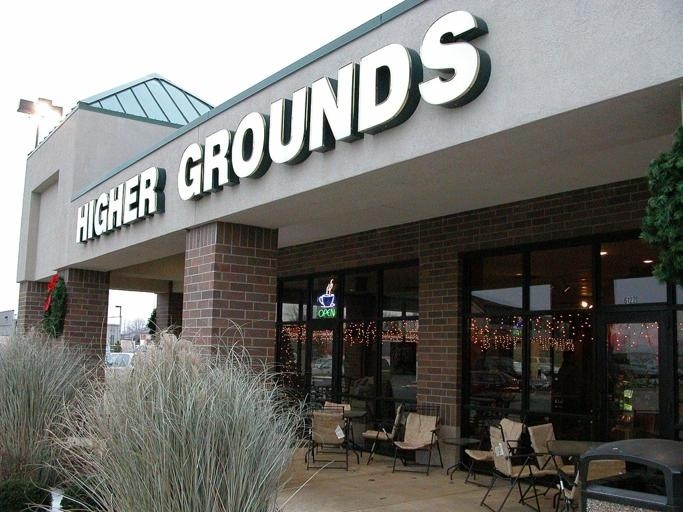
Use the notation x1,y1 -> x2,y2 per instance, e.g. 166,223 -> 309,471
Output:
465,352 -> 560,410
614,353 -> 657,389
284,350 -> 391,402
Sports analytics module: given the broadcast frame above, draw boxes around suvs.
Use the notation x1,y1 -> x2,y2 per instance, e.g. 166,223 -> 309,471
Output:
106,350 -> 134,381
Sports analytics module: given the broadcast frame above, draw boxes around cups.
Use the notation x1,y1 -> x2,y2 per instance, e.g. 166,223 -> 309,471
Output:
319,294 -> 335,306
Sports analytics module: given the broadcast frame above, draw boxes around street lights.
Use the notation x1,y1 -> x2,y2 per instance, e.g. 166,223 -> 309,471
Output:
115,305 -> 123,340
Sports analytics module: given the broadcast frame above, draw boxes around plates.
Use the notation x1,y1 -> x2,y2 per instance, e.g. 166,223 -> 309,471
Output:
320,306 -> 334,309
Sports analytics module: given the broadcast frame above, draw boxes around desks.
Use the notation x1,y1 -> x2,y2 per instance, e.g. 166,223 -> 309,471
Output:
443,437 -> 481,480
547,446 -> 590,502
306,410 -> 367,465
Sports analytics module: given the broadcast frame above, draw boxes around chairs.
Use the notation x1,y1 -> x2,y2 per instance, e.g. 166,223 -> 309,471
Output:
319,400 -> 362,465
390,405 -> 443,477
363,401 -> 405,465
554,457 -> 622,510
464,418 -> 526,486
306,405 -> 349,471
518,422 -> 575,503
480,423 -> 557,510
